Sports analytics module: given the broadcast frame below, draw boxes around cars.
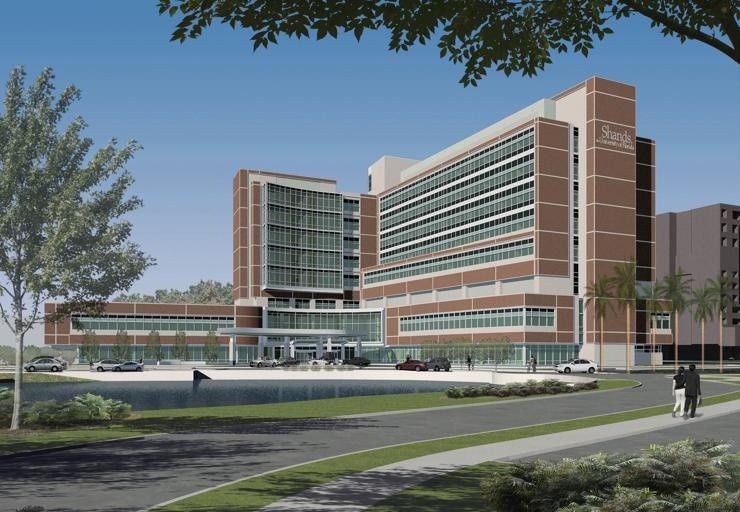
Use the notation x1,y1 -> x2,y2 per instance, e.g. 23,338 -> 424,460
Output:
554,358 -> 598,374
308,357 -> 336,366
90,358 -> 144,372
24,355 -> 68,372
249,356 -> 300,368
395,357 -> 451,372
343,357 -> 371,366
